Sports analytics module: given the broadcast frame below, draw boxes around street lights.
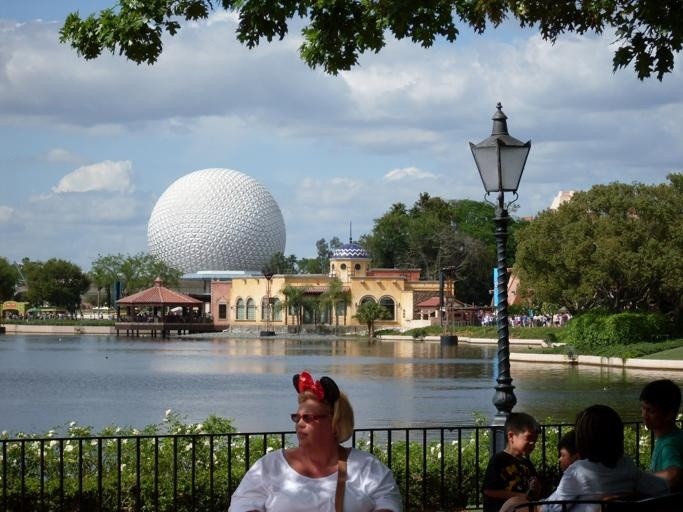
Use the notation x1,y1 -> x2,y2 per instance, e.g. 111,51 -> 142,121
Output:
468,102 -> 531,460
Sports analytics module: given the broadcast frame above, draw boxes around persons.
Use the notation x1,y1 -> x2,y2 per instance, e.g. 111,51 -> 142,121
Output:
6,308 -> 213,323
481,380 -> 683,512
228,371 -> 404,512
477,310 -> 573,328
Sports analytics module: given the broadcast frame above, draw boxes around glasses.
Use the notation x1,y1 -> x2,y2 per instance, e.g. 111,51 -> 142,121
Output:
291,413 -> 330,422
